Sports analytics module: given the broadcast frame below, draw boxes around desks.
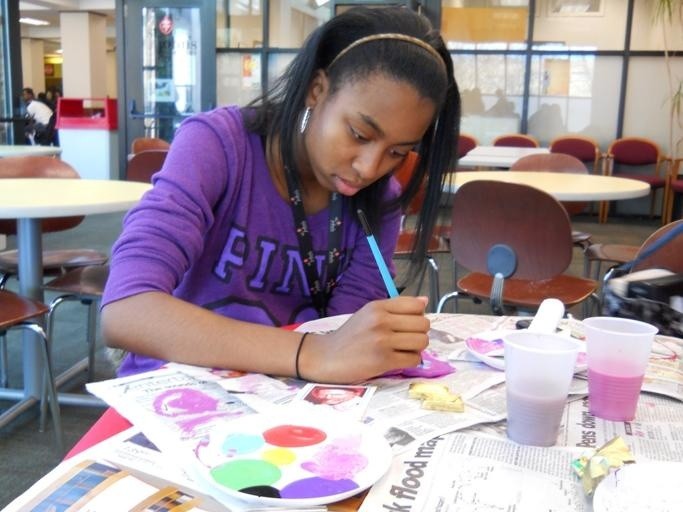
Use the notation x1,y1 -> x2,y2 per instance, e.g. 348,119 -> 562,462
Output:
2,179 -> 155,431
424,170 -> 652,203
54,317 -> 682,512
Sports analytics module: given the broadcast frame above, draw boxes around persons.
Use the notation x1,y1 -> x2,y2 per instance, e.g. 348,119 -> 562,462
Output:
10,83 -> 58,147
90,5 -> 463,386
310,386 -> 363,408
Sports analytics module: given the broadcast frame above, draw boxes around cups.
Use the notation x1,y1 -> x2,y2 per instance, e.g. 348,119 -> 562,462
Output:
501,329 -> 582,448
579,316 -> 659,423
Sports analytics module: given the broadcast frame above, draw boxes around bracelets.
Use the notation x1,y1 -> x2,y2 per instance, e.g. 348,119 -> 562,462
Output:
292,331 -> 310,381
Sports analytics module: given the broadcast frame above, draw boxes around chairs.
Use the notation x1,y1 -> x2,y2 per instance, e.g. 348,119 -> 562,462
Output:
1,134 -> 171,451
392,133 -> 683,317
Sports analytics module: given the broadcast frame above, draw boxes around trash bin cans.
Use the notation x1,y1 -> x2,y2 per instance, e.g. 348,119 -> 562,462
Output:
55,96 -> 120,180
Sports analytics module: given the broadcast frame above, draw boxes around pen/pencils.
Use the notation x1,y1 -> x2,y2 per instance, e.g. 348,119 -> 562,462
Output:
357,209 -> 424,366
